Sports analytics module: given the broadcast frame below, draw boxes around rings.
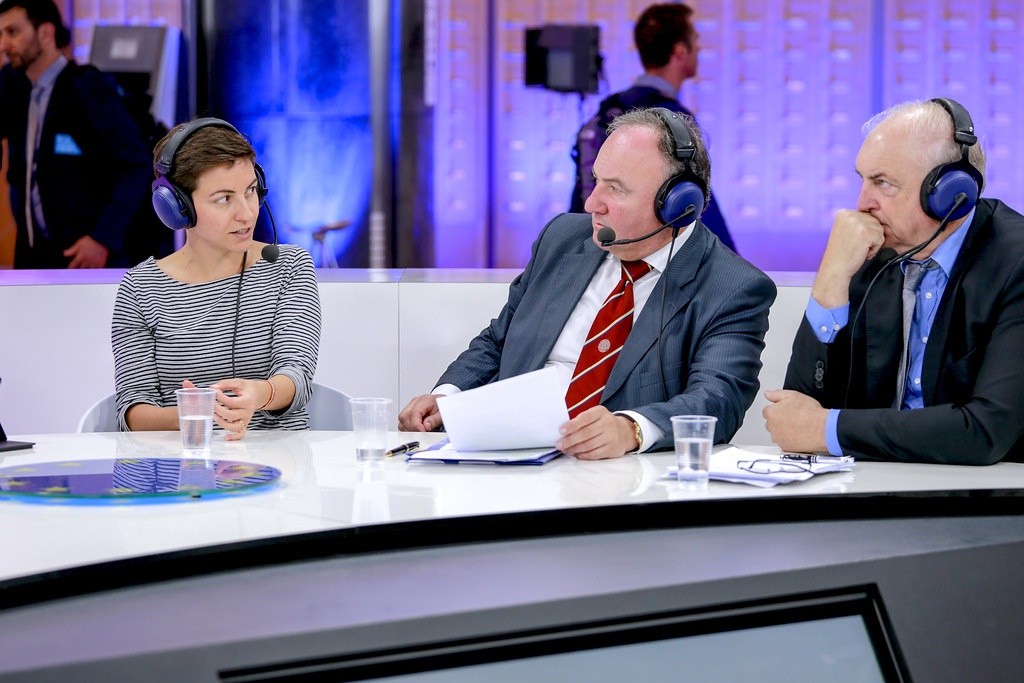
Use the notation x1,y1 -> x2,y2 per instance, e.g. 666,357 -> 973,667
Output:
240,420 -> 245,427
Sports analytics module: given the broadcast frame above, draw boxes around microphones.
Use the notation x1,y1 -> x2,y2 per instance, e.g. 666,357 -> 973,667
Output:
261,200 -> 279,263
597,204 -> 695,246
880,193 -> 968,266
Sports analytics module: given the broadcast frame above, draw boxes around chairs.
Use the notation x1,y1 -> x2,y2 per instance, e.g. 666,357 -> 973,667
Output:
77,381 -> 364,434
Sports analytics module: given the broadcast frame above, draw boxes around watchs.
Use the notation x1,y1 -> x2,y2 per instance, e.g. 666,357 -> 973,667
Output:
614,414 -> 643,449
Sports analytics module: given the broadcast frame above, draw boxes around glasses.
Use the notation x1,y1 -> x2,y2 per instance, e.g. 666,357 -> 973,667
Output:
737,457 -> 816,478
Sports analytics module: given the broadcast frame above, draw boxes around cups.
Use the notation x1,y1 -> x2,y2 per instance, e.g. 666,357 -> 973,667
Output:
350,398 -> 393,461
178,459 -> 216,491
175,388 -> 218,450
669,415 -> 718,486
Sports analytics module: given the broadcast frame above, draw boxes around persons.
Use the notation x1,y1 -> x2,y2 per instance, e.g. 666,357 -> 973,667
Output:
762,97 -> 1024,466
0,0 -> 155,269
111,118 -> 322,440
398,107 -> 777,461
566,2 -> 739,254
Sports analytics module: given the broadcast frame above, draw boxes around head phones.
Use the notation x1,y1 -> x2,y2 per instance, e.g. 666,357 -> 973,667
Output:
152,117 -> 268,230
919,98 -> 984,221
645,108 -> 708,229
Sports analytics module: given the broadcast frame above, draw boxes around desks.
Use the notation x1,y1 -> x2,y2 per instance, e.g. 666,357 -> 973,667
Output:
1,428 -> 1024,683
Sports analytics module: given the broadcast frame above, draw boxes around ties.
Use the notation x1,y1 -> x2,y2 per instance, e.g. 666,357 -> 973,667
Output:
564,257 -> 653,424
891,259 -> 939,413
26,87 -> 40,246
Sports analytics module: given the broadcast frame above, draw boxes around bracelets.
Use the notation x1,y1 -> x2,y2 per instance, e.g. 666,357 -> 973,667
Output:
256,380 -> 276,411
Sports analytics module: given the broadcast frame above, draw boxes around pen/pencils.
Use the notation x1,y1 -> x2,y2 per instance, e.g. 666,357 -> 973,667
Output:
781,453 -> 842,464
386,440 -> 420,457
